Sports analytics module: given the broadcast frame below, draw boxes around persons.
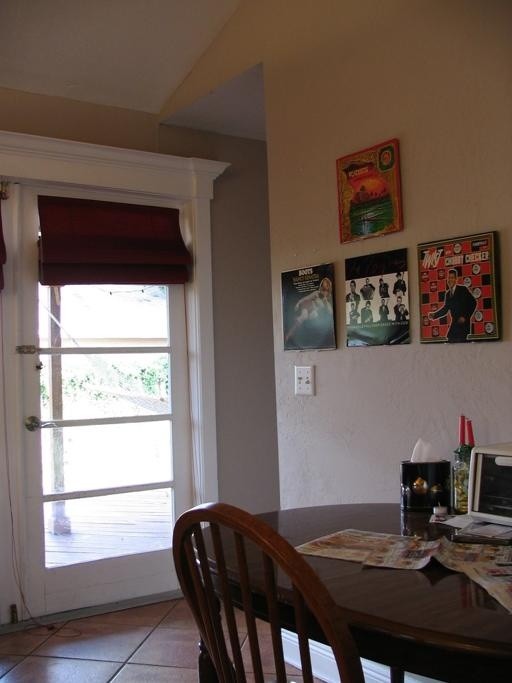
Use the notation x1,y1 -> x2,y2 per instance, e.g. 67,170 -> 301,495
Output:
346,271 -> 409,324
428,267 -> 478,340
285,276 -> 334,347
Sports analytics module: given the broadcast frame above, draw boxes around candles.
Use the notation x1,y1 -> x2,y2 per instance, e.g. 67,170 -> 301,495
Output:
457,413 -> 475,448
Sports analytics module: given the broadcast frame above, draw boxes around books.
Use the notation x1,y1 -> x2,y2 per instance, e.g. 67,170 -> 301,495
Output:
450,522 -> 511,545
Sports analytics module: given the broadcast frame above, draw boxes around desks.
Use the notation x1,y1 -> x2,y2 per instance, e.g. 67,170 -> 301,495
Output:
188,501 -> 510,681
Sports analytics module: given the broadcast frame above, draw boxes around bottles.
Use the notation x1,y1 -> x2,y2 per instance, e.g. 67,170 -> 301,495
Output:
452,442 -> 474,513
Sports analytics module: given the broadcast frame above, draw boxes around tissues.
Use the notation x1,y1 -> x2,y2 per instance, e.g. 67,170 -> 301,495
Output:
400,521 -> 452,589
401,437 -> 451,518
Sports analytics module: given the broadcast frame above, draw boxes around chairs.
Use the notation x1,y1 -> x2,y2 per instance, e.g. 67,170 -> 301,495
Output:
171,503 -> 365,683
